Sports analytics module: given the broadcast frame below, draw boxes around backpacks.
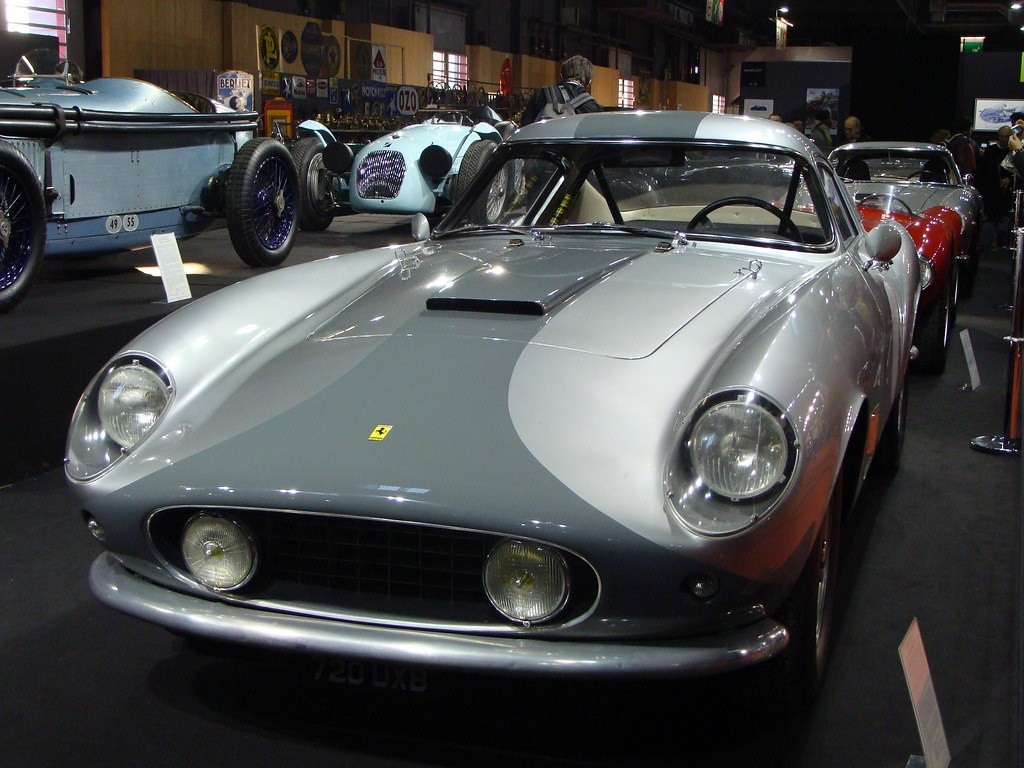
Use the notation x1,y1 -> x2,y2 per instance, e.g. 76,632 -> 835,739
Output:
522,86 -> 595,190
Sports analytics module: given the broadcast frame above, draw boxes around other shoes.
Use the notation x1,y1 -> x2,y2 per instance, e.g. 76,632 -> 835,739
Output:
968,248 -> 980,256
1001,246 -> 1010,258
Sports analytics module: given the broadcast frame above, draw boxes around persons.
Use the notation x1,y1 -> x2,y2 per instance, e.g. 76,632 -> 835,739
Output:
932,112 -> 1024,255
521,55 -> 602,221
768,106 -> 872,158
56,61 -> 84,84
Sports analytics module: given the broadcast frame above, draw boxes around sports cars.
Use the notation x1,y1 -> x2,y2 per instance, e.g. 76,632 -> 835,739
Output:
0,46 -> 304,312
61,107 -> 923,694
764,187 -> 963,376
780,139 -> 983,255
291,100 -> 527,233
